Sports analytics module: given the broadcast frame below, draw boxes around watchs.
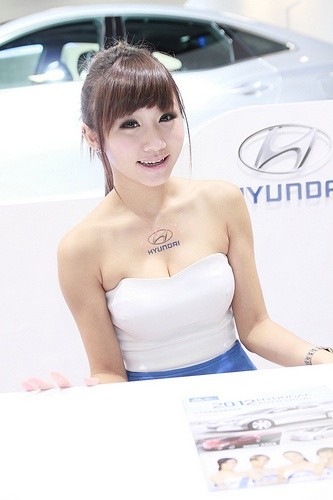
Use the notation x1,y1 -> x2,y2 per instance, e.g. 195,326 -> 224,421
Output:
303,346 -> 333,366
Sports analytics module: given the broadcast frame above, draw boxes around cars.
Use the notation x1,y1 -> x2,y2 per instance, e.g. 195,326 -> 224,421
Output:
1,4 -> 333,202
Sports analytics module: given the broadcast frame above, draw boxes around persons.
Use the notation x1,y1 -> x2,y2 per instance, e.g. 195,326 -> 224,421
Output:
204,447 -> 333,493
22,40 -> 333,392
